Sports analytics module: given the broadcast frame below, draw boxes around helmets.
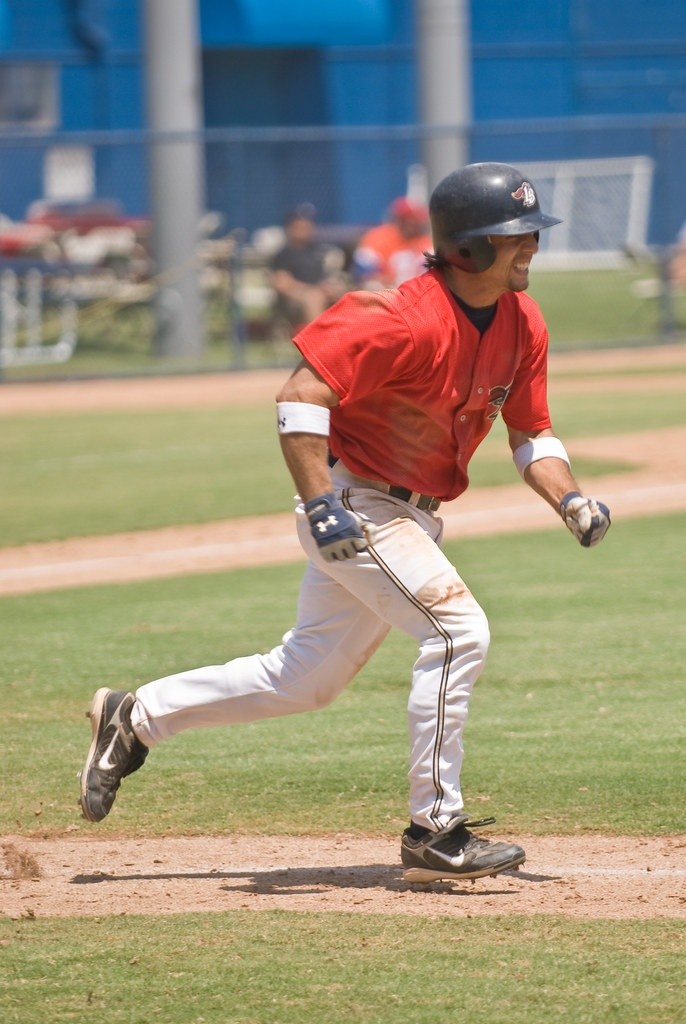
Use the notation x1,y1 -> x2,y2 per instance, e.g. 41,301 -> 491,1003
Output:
429,162 -> 564,273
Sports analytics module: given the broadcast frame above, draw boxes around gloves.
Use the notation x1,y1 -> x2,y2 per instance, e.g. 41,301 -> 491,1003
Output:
560,492 -> 611,547
304,493 -> 369,563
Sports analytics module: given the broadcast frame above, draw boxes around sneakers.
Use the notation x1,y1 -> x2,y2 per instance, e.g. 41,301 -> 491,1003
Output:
77,687 -> 149,822
401,815 -> 526,885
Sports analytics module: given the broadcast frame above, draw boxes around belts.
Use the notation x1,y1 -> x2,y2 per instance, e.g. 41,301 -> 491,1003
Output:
328,455 -> 442,512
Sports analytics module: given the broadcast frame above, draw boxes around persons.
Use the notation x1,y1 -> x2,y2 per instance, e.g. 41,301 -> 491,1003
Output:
270,200 -> 438,350
79,160 -> 610,887
